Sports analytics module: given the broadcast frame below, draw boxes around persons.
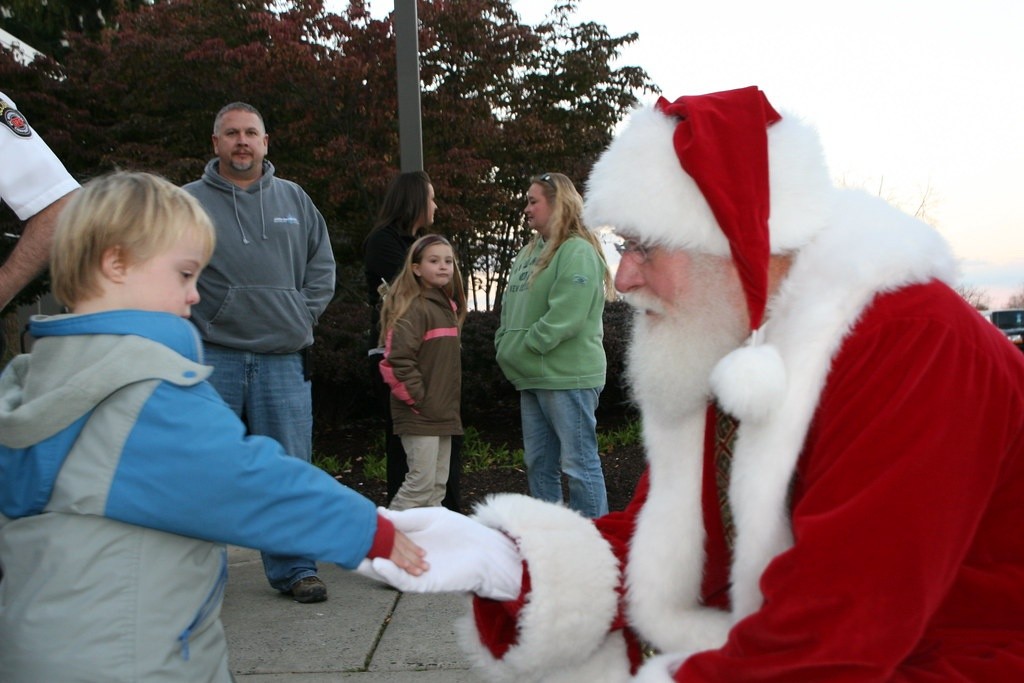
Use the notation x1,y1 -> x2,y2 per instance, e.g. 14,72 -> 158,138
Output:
0,167 -> 431,682
359,168 -> 465,513
494,173 -> 611,518
0,91 -> 81,308
380,235 -> 468,510
350,85 -> 1024,683
176,102 -> 338,603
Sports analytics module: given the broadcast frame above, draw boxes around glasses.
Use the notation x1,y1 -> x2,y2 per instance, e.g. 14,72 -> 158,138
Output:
613,239 -> 649,265
540,173 -> 555,189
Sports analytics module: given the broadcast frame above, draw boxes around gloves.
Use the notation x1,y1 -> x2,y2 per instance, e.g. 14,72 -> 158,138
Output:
350,506 -> 525,603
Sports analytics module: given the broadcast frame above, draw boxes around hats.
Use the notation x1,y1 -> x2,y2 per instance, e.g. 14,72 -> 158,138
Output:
581,85 -> 839,426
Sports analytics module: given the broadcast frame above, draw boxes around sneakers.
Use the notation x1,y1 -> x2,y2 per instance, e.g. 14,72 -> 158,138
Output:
293,576 -> 327,602
